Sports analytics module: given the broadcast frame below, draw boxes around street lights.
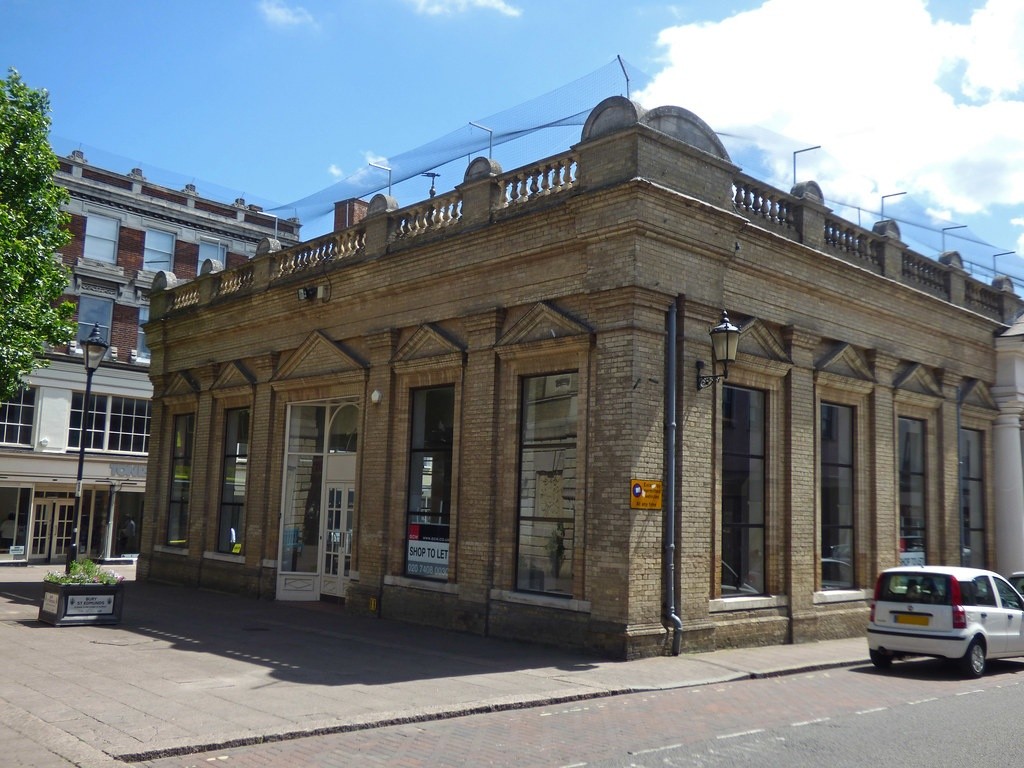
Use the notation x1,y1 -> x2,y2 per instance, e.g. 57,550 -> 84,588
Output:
64,322 -> 112,573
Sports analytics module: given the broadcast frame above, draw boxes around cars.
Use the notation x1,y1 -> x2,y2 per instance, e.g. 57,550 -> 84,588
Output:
867,565 -> 1024,677
721,561 -> 759,598
821,557 -> 852,589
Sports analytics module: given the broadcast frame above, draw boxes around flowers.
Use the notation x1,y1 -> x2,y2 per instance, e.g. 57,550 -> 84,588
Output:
44,560 -> 125,586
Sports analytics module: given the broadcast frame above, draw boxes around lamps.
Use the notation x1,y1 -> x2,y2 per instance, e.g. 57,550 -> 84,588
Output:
695,309 -> 741,391
735,241 -> 741,251
297,286 -> 315,300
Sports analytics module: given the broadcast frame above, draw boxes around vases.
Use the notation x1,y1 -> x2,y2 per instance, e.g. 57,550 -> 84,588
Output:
38,580 -> 124,625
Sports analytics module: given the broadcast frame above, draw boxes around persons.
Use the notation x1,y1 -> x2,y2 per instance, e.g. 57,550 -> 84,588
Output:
120,514 -> 134,551
0,512 -> 16,549
905,579 -> 918,593
